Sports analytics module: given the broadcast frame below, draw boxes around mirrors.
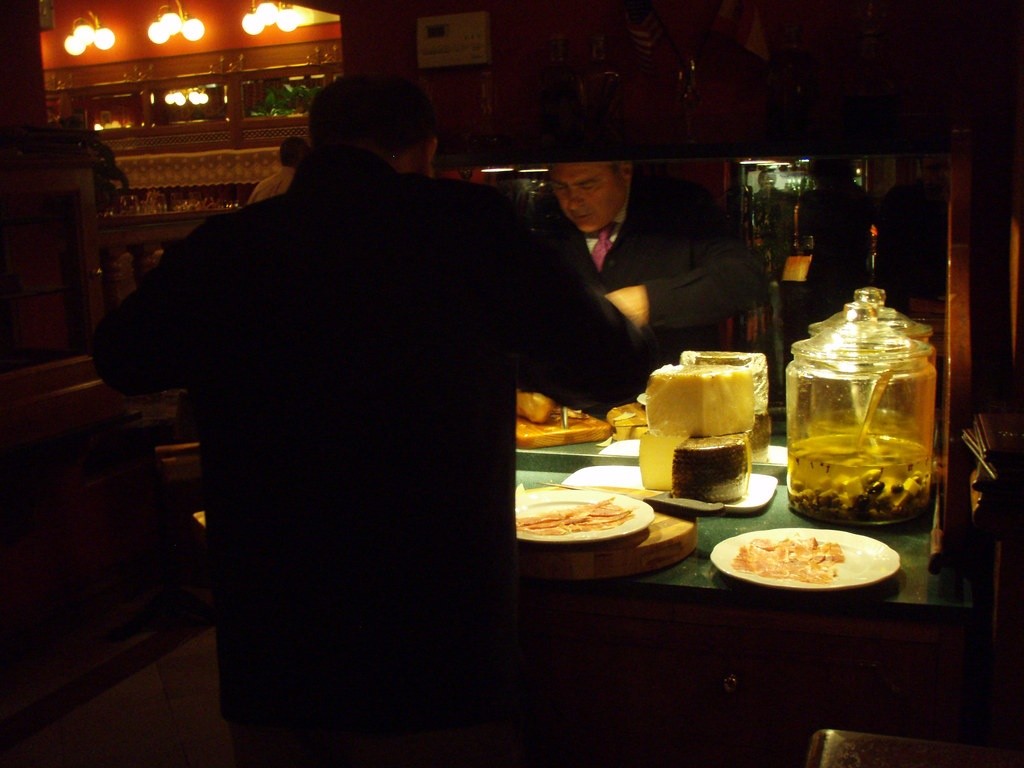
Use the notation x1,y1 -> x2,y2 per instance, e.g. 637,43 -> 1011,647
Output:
44,73 -> 326,130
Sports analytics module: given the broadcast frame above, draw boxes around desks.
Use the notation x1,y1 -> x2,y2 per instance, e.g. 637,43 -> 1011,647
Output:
190,418 -> 982,768
98,197 -> 235,312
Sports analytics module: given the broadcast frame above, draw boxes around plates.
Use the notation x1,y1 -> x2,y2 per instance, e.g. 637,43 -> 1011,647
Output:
561,465 -> 779,514
515,490 -> 655,543
598,440 -> 790,470
709,527 -> 900,590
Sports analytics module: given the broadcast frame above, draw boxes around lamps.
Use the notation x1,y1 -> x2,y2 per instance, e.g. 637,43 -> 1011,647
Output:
241,0 -> 298,36
166,85 -> 209,105
63,0 -> 115,55
147,0 -> 205,45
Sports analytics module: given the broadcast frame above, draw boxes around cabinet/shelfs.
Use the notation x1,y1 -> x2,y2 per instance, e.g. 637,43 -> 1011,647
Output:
0,149 -> 127,406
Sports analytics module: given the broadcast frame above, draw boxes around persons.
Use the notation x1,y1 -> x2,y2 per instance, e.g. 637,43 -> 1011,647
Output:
248,136 -> 310,205
93,72 -> 658,768
525,159 -> 952,421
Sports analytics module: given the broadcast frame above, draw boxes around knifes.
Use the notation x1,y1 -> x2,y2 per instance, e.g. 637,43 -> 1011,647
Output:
538,480 -> 725,515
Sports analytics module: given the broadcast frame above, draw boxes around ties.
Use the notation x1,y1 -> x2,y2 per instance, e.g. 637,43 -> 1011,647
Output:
590,221 -> 617,272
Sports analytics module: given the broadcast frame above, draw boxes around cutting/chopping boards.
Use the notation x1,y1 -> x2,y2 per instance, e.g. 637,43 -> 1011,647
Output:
516,407 -> 612,448
516,486 -> 703,581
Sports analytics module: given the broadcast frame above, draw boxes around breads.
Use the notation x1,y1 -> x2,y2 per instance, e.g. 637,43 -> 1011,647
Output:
517,391 -> 553,422
638,350 -> 772,502
